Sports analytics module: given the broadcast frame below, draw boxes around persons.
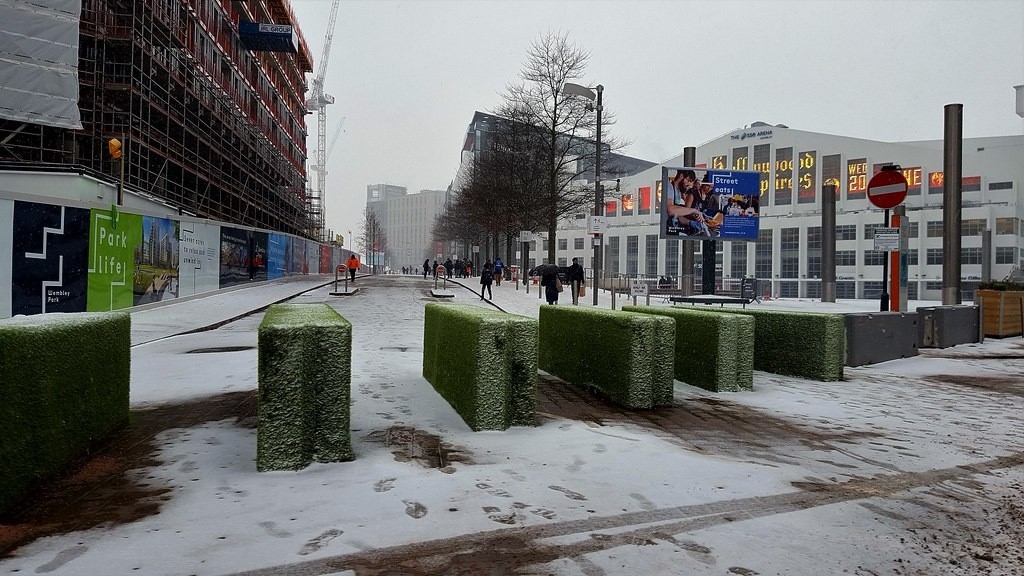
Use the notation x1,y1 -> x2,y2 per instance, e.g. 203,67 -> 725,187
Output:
347,255 -> 359,283
567,258 -> 584,305
415,268 -> 418,273
402,265 -> 413,274
423,259 -> 429,279
659,276 -> 672,289
494,257 -> 503,286
667,170 -> 745,238
481,263 -> 493,300
433,257 -> 473,280
487,259 -> 492,278
541,257 -> 560,305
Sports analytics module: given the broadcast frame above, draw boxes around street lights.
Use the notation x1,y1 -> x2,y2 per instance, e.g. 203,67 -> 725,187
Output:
562,81 -> 608,306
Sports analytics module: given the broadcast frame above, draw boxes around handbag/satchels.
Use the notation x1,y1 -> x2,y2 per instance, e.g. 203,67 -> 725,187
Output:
580,283 -> 585,297
556,277 -> 564,292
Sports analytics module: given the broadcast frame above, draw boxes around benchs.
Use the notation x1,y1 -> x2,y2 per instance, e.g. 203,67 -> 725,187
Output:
669,297 -> 750,309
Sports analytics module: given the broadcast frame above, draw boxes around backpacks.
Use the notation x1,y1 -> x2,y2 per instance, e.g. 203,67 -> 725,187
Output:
483,273 -> 493,283
496,260 -> 502,270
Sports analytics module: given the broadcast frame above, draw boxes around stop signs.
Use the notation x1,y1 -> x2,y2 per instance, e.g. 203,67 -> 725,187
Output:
337,265 -> 345,273
438,266 -> 443,273
866,168 -> 908,209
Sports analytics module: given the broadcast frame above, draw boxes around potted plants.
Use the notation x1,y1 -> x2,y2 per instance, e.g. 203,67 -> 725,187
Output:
976,279 -> 1024,340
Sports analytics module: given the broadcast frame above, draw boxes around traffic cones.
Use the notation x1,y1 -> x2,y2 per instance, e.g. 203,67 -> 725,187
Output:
532,274 -> 538,285
512,269 -> 518,282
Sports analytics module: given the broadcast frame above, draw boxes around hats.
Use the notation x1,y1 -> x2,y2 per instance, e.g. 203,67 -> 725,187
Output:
572,258 -> 578,261
351,254 -> 355,258
484,262 -> 489,268
